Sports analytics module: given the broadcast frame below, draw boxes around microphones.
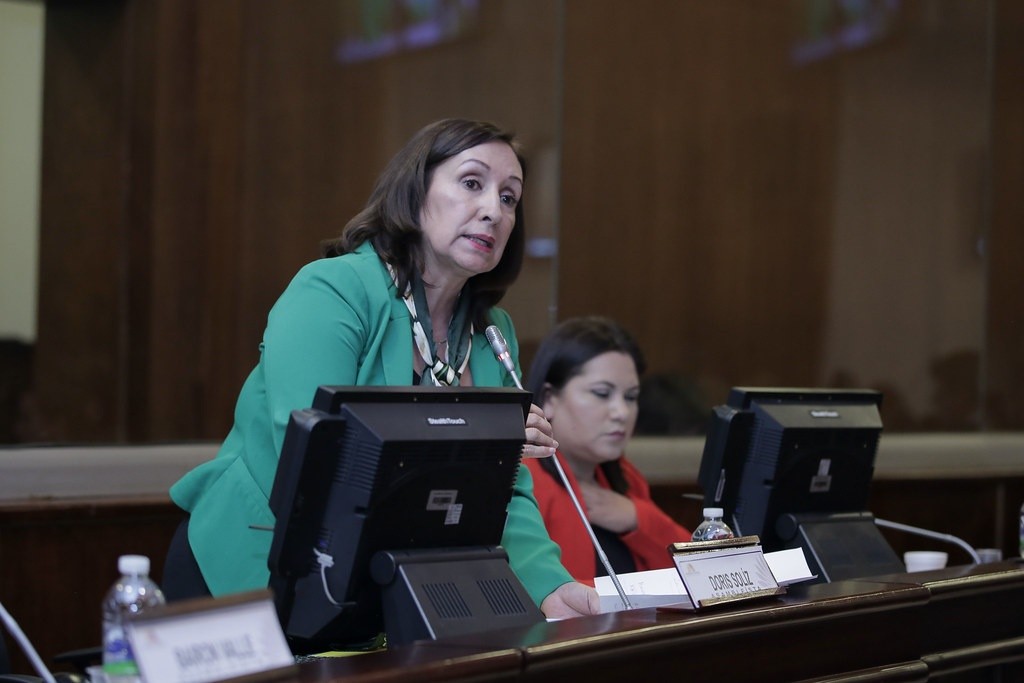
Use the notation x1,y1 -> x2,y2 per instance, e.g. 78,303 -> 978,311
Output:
486,325 -> 632,612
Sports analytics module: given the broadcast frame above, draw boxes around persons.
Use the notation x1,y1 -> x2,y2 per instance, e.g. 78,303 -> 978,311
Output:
522,316 -> 693,588
160,118 -> 603,630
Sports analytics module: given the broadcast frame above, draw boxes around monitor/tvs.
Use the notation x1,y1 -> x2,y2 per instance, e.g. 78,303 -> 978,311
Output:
694,384 -> 885,555
266,384 -> 532,653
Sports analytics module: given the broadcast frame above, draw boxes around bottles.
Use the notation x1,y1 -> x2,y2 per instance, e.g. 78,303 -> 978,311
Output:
103,555 -> 165,683
693,508 -> 734,542
